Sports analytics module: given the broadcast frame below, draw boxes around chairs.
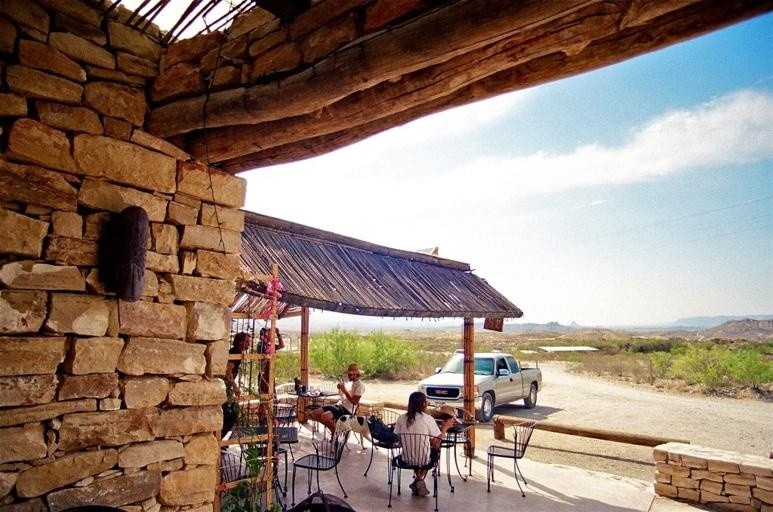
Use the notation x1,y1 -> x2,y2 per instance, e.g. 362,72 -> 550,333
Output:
222,377 -> 477,509
486,421 -> 536,497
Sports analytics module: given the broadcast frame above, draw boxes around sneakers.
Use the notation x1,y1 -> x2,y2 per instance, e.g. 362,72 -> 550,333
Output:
409,480 -> 429,496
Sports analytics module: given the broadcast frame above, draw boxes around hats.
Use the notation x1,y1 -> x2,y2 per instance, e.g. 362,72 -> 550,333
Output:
343,364 -> 360,374
435,405 -> 462,425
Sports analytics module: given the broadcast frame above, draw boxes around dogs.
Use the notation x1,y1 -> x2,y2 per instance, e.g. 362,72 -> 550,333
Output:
334,414 -> 378,451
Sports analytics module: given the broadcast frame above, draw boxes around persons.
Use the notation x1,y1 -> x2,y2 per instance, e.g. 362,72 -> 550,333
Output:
256,327 -> 285,426
222,331 -> 250,438
393,390 -> 457,497
309,364 -> 366,442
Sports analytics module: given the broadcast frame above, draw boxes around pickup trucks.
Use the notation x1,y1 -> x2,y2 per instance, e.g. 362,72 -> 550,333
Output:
418,352 -> 541,422
